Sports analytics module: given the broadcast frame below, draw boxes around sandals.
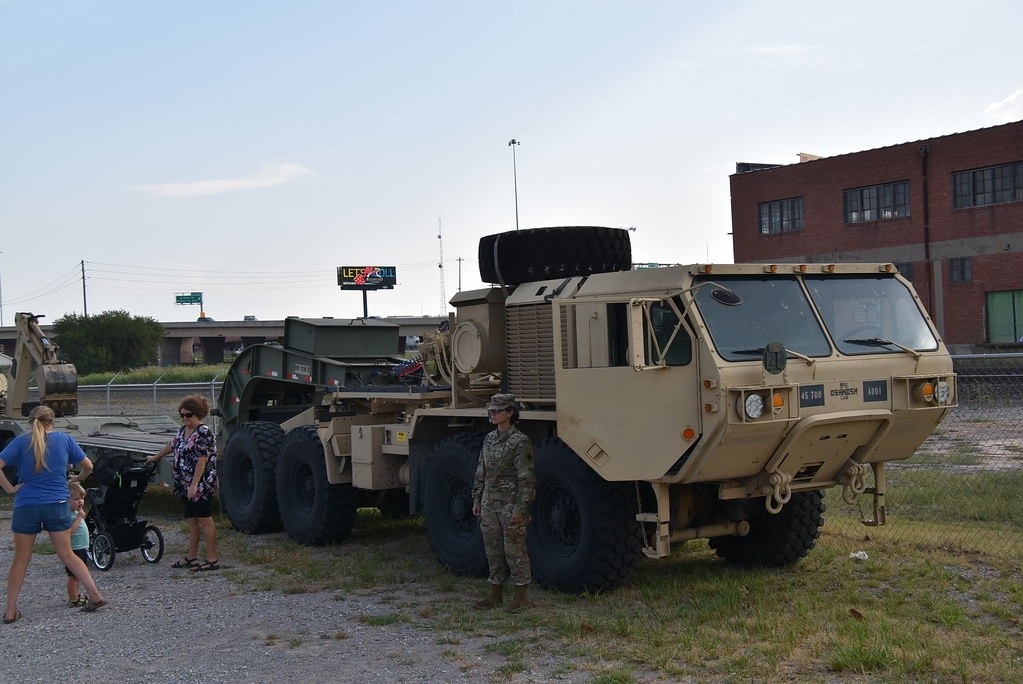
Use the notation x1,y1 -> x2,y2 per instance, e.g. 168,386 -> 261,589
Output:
68,594 -> 89,608
172,556 -> 199,568
190,559 -> 220,572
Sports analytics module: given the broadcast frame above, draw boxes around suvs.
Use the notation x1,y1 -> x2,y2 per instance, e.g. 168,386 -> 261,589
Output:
234,348 -> 243,355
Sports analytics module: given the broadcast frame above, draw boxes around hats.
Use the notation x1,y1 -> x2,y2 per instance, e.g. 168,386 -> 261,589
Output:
487,394 -> 515,410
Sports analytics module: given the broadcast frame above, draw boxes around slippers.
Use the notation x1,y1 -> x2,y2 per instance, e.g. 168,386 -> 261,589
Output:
3,610 -> 22,624
79,599 -> 108,612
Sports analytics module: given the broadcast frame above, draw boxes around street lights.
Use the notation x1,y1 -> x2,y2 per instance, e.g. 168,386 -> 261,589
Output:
455,257 -> 464,292
508,139 -> 521,230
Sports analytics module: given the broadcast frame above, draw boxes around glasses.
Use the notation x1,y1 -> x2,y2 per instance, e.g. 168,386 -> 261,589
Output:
180,413 -> 195,418
489,408 -> 511,414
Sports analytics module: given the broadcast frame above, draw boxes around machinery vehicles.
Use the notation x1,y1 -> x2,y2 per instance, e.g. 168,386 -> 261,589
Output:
210,225 -> 959,595
0,311 -> 140,490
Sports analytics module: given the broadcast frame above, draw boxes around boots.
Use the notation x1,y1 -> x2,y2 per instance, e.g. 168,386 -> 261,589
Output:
505,584 -> 531,613
475,584 -> 504,609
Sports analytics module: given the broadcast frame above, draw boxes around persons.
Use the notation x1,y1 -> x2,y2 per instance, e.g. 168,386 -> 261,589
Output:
471,394 -> 536,613
65,484 -> 89,607
0,406 -> 106,621
146,398 -> 220,571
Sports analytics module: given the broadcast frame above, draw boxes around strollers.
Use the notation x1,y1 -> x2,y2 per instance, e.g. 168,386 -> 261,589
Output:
85,450 -> 164,571
